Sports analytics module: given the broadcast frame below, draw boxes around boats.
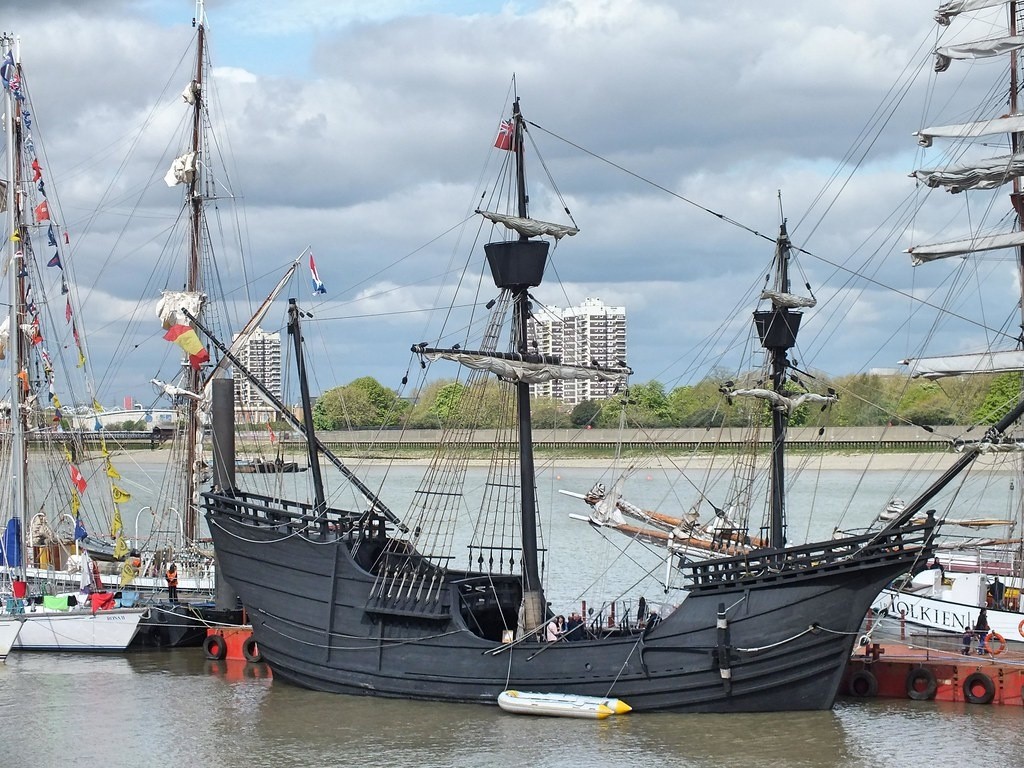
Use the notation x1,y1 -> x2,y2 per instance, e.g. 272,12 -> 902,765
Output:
195,455 -> 306,473
496,688 -> 632,720
199,93 -> 1023,712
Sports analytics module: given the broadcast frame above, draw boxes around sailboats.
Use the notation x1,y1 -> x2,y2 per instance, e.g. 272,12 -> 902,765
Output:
0,31 -> 244,653
558,0 -> 1022,641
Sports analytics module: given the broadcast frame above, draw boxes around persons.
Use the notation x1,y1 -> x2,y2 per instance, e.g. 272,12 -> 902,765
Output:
542,606 -> 586,645
961,626 -> 974,655
929,556 -> 944,583
636,596 -> 649,629
164,563 -> 179,602
975,608 -> 990,655
990,577 -> 1007,609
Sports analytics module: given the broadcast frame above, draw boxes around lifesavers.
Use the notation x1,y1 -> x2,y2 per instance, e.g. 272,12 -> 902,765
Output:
203,635 -> 227,661
1018,620 -> 1024,637
906,667 -> 938,701
242,637 -> 264,663
984,633 -> 1006,655
962,672 -> 996,705
848,670 -> 878,699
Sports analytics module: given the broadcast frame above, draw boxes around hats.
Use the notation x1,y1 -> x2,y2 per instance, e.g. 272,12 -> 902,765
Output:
935,557 -> 938,559
555,615 -> 564,619
980,608 -> 986,614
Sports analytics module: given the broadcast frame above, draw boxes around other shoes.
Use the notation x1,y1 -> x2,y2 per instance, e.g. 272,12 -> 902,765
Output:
976,650 -> 983,655
961,649 -> 971,656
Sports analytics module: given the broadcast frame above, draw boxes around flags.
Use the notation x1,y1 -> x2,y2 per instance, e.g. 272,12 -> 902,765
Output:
162,324 -> 211,371
41,359 -> 138,588
494,119 -> 525,152
0,49 -> 86,353
309,251 -> 327,297
